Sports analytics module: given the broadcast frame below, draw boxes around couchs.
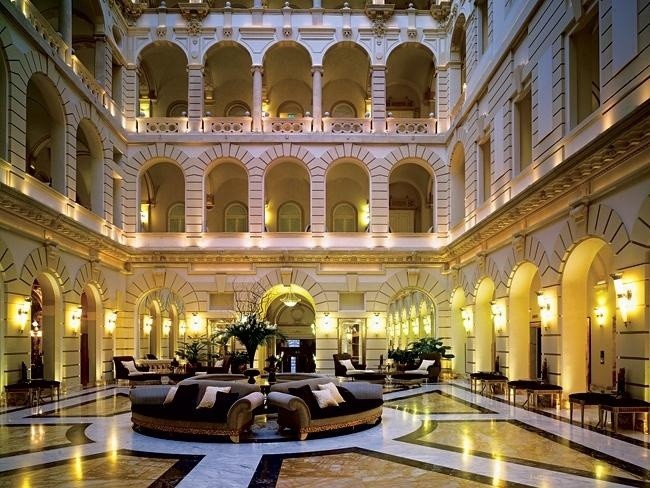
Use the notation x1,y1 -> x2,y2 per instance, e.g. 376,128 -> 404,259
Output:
113,352 -> 443,444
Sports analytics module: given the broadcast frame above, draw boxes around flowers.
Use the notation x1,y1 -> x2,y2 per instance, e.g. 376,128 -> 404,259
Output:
209,309 -> 288,368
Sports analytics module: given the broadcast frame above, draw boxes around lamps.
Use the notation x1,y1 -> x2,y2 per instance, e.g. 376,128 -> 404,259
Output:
458,271 -> 635,335
12,294 -> 201,335
279,286 -> 301,307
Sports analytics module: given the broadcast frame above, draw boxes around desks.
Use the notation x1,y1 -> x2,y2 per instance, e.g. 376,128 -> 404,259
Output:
3,378 -> 62,409
468,367 -> 650,439
